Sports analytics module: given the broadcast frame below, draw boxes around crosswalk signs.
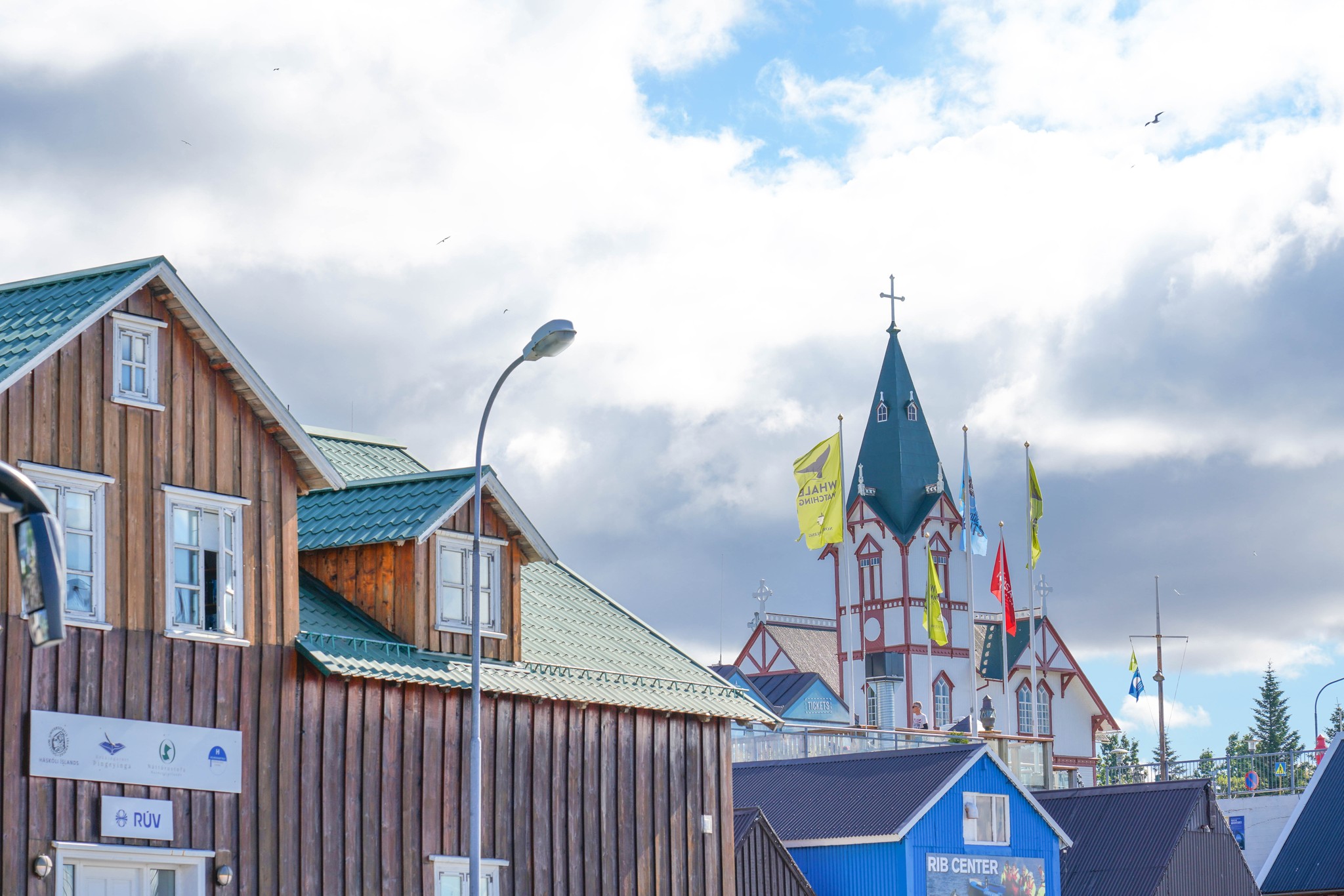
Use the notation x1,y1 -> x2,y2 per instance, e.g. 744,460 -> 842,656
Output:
1276,762 -> 1286,777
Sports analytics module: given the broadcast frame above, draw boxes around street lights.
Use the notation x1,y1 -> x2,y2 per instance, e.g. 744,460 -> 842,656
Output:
1242,738 -> 1262,797
466,318 -> 578,896
1109,749 -> 1130,785
1153,668 -> 1166,781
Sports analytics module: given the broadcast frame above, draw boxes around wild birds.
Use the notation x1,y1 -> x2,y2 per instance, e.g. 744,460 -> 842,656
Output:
1144,111 -> 1165,126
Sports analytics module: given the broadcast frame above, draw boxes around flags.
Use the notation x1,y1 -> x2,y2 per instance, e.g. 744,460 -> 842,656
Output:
1129,650 -> 1138,672
959,429 -> 987,556
1129,668 -> 1144,702
922,544 -> 948,646
1025,457 -> 1045,570
990,535 -> 1017,638
794,431 -> 844,550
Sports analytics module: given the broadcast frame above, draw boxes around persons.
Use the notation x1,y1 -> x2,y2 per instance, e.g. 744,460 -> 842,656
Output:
912,701 -> 928,744
